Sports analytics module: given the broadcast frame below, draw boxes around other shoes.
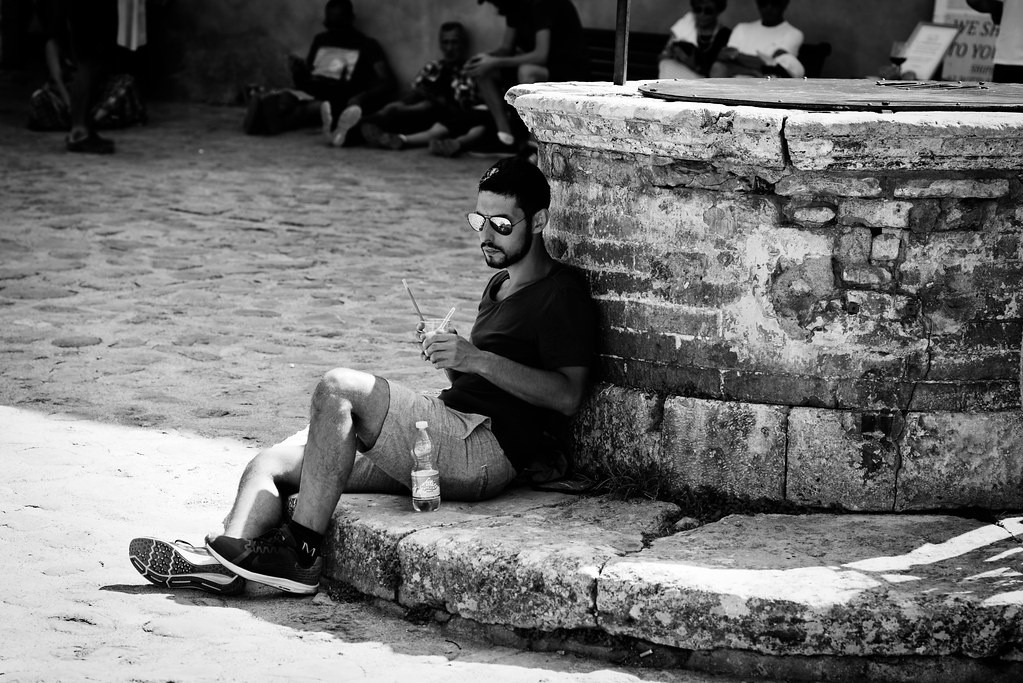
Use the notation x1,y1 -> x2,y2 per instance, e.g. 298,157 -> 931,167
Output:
430,138 -> 460,158
334,105 -> 362,147
321,101 -> 335,144
380,133 -> 406,151
67,131 -> 115,153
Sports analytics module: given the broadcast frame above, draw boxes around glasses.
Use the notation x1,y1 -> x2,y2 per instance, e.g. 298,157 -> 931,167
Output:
465,211 -> 537,236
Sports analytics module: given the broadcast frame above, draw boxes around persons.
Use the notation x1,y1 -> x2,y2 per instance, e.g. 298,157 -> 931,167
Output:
239,0 -> 590,155
655,0 -> 806,79
966,0 -> 1023,83
127,157 -> 594,599
21,0 -> 151,155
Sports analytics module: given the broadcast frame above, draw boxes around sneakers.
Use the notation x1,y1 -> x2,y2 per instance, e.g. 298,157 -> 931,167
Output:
128,536 -> 247,598
205,526 -> 323,595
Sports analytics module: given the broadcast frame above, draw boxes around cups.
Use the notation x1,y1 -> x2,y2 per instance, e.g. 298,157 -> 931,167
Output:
420,319 -> 451,332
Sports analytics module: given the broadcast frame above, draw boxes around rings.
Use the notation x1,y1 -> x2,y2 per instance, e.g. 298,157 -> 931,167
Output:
424,350 -> 430,357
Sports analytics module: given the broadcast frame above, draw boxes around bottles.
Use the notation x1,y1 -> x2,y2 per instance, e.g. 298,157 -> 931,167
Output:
411,421 -> 441,513
